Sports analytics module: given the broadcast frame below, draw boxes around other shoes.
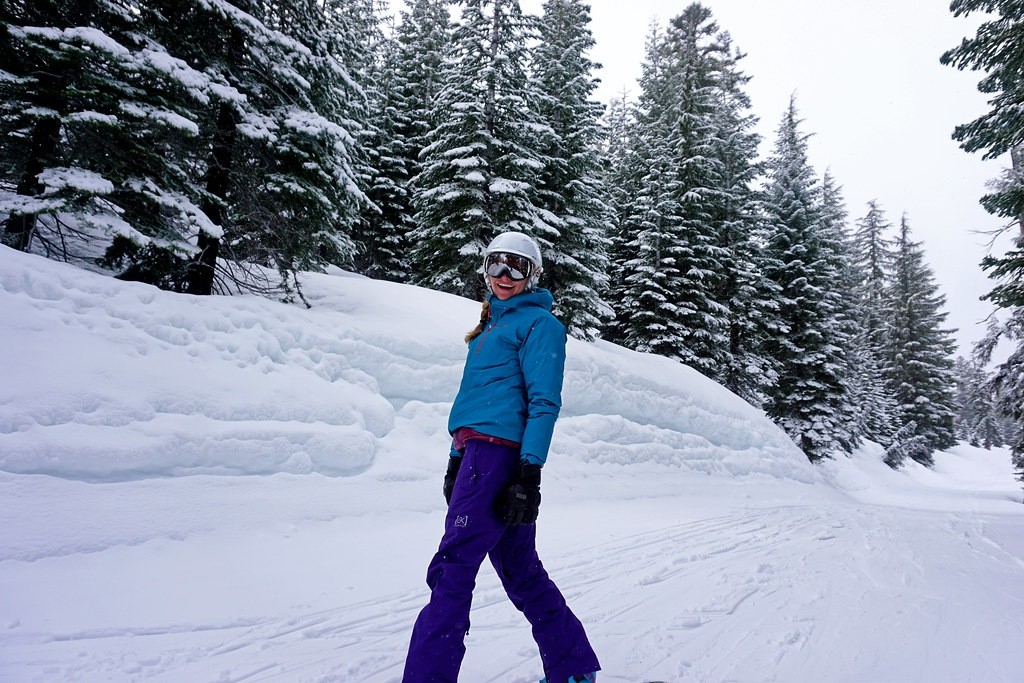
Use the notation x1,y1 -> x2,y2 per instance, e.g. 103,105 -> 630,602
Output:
538,671 -> 596,683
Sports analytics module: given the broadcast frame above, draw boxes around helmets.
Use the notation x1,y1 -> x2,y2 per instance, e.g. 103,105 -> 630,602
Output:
483,231 -> 542,292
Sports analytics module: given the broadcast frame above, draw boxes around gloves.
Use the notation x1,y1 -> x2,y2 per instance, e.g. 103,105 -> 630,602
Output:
444,456 -> 461,507
501,463 -> 541,526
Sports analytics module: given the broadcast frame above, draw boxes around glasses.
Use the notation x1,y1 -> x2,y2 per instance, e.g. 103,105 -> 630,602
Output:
485,252 -> 534,281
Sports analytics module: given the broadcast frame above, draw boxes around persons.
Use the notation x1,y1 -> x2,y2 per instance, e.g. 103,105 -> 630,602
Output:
402,232 -> 602,683
